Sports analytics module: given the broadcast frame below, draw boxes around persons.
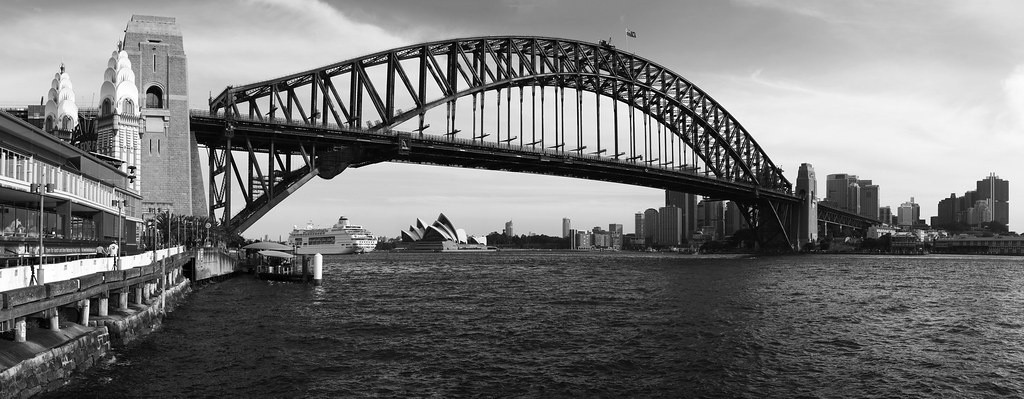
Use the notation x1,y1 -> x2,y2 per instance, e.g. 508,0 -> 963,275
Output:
96,240 -> 119,258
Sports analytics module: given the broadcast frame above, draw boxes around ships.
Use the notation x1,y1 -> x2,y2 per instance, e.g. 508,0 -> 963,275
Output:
287,215 -> 378,255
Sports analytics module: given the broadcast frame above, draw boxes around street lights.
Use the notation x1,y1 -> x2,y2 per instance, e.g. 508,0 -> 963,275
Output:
29,181 -> 53,284
149,207 -> 161,263
112,199 -> 129,273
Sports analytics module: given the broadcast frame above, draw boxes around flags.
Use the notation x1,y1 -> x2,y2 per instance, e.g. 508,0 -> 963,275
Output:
627,28 -> 636,38
